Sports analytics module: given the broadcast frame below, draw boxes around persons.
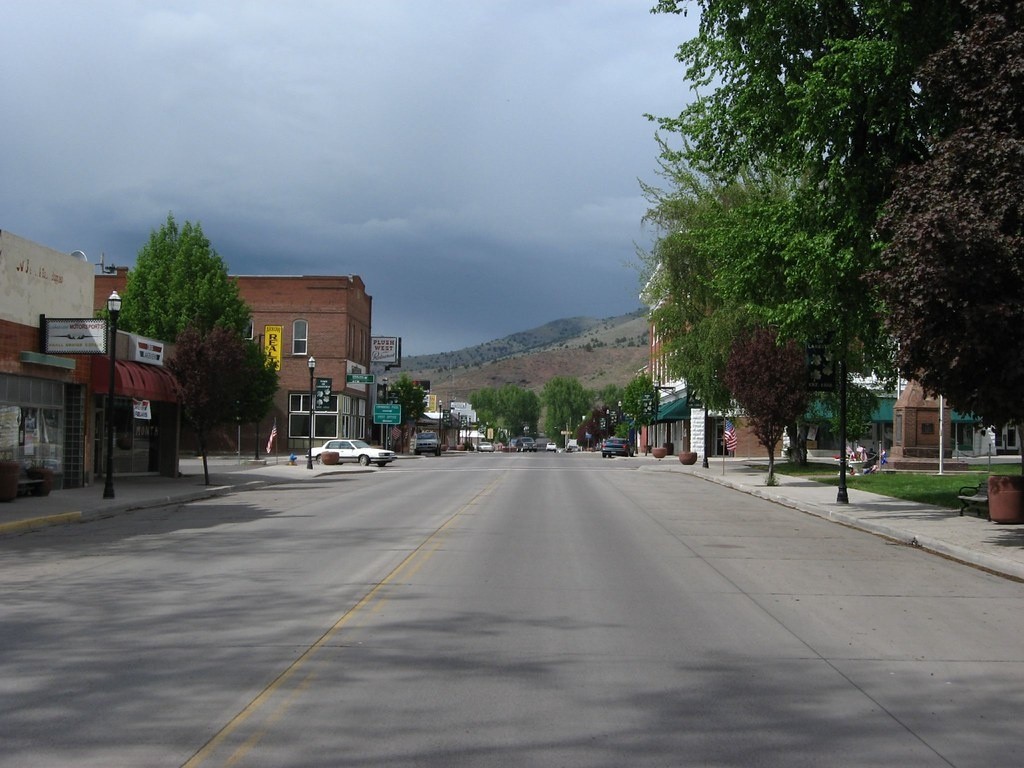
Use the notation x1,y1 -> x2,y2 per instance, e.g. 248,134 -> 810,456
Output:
847,445 -> 888,468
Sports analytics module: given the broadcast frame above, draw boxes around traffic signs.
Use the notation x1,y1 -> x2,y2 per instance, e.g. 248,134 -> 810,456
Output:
373,404 -> 401,413
374,414 -> 401,425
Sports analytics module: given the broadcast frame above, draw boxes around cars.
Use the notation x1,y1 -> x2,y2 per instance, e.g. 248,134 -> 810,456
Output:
478,441 -> 495,452
602,438 -> 635,458
305,439 -> 397,468
516,437 -> 538,452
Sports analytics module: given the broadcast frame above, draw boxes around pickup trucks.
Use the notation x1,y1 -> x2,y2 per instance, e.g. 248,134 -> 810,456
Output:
414,432 -> 442,456
546,442 -> 557,452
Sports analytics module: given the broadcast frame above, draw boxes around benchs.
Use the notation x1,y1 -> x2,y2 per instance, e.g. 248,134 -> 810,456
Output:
958,481 -> 988,519
16,469 -> 48,499
865,453 -> 877,468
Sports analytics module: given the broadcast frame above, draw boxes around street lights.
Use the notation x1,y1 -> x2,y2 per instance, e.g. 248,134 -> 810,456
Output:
102,290 -> 122,498
653,379 -> 660,447
307,354 -> 316,470
618,401 -> 622,425
606,409 -> 610,437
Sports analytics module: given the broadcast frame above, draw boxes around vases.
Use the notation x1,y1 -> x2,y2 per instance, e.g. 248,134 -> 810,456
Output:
457,444 -> 464,450
441,444 -> 448,450
322,452 -> 339,465
652,448 -> 667,458
679,452 -> 697,466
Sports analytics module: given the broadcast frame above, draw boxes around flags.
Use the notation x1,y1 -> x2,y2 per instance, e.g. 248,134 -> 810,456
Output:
266,423 -> 277,453
646,397 -> 690,426
725,420 -> 737,451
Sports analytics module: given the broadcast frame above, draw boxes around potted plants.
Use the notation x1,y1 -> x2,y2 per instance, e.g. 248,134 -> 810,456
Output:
0,450 -> 21,503
26,460 -> 53,496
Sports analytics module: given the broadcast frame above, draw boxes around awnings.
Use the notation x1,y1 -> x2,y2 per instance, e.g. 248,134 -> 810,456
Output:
804,398 -> 897,423
92,355 -> 185,404
415,414 -> 437,425
951,411 -> 983,423
